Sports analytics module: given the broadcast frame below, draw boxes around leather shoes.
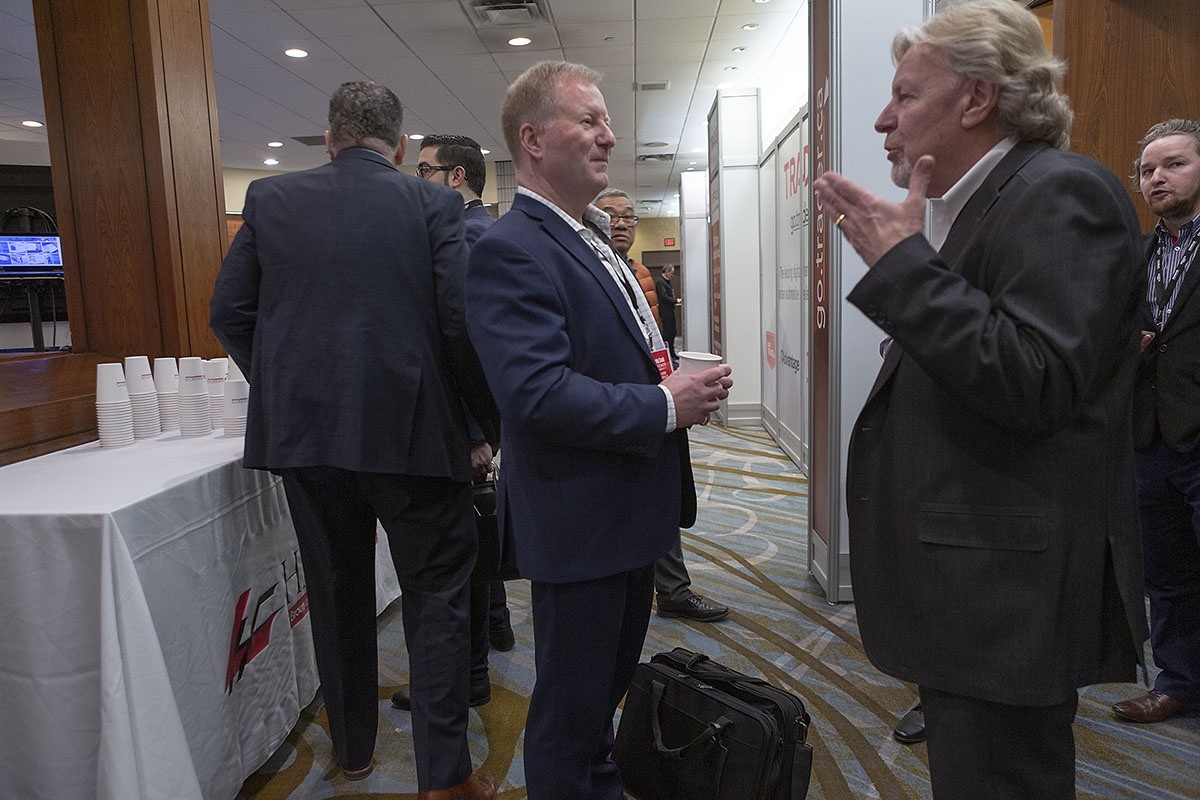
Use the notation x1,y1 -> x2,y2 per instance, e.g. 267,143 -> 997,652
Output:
490,627 -> 515,652
391,674 -> 491,709
657,594 -> 730,622
1113,688 -> 1200,723
893,703 -> 927,742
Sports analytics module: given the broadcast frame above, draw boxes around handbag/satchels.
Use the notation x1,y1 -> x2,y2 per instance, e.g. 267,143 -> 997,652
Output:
609,647 -> 813,800
470,461 -> 524,583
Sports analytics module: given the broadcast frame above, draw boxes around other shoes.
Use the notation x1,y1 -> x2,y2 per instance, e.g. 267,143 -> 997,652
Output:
417,773 -> 497,800
341,755 -> 373,780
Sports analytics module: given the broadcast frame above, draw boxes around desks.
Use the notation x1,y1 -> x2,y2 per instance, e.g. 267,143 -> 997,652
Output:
0,430 -> 409,800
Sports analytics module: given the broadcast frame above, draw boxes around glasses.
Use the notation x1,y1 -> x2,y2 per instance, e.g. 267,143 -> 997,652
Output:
607,215 -> 639,227
415,166 -> 468,182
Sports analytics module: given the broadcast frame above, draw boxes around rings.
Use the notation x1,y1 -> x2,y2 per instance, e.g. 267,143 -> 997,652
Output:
835,213 -> 845,227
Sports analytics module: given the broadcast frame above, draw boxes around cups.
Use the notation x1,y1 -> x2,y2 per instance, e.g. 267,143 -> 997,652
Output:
153,358 -> 180,430
177,356 -> 213,438
227,356 -> 250,394
224,380 -> 249,438
95,363 -> 136,448
677,351 -> 724,374
124,355 -> 162,440
202,357 -> 230,429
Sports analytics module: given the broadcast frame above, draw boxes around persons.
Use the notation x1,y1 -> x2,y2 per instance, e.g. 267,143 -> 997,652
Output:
390,134 -> 730,712
892,117 -> 1200,742
210,81 -> 500,800
455,63 -> 734,800
808,0 -> 1140,800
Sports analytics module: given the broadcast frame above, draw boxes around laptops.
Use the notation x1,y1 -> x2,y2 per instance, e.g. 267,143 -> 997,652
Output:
0,232 -> 64,277
